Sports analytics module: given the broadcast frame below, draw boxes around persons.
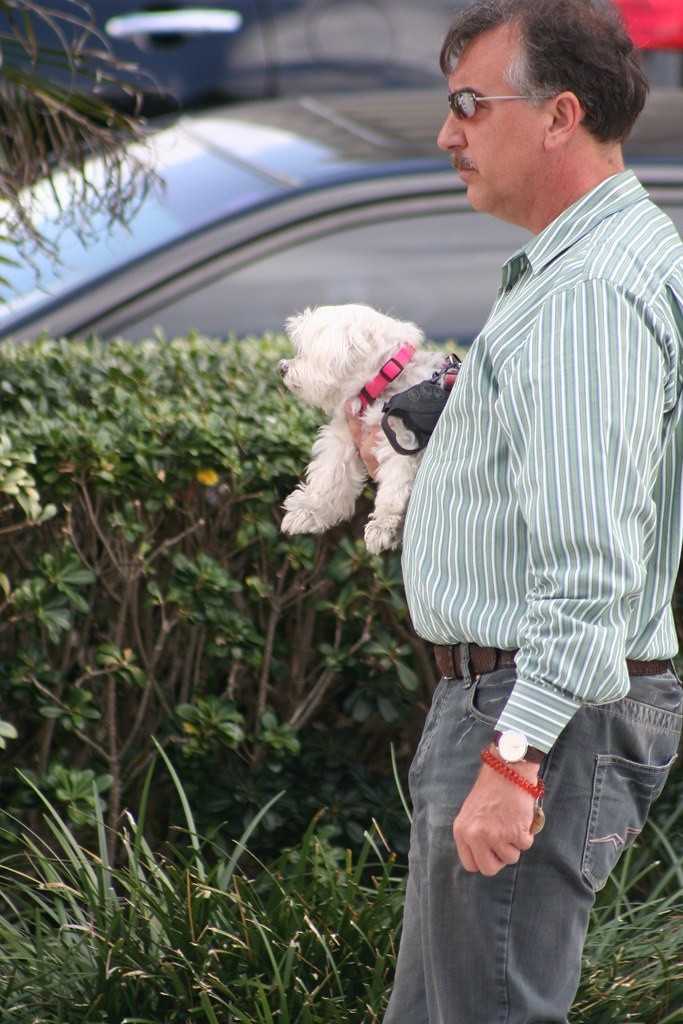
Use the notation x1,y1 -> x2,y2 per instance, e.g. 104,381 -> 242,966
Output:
346,0 -> 683,1024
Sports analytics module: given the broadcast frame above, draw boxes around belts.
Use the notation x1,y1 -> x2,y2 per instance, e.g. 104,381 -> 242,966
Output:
433,641 -> 669,680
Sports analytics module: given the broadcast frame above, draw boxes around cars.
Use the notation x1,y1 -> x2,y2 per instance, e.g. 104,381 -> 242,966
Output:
0,81 -> 683,356
1,0 -> 683,166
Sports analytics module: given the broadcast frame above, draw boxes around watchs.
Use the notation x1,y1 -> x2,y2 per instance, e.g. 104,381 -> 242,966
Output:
492,730 -> 547,765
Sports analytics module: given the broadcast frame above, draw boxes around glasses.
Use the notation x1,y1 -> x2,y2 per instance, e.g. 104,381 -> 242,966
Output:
445,86 -> 561,120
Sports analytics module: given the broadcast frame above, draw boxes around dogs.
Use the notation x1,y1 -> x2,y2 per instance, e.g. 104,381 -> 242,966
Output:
275,302 -> 463,558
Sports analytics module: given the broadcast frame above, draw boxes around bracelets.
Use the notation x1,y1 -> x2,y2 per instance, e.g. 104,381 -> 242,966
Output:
479,749 -> 545,799
